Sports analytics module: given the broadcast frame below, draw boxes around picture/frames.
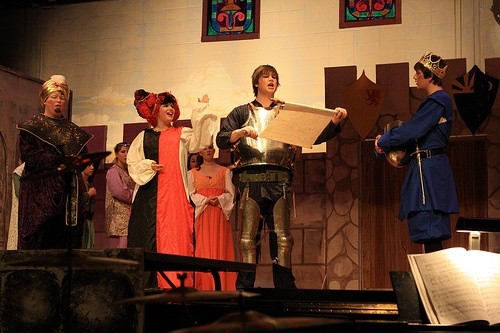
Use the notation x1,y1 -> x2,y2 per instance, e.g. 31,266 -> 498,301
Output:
201,0 -> 260,42
339,0 -> 401,28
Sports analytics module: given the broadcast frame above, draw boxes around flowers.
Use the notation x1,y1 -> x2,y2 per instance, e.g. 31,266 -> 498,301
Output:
139,95 -> 156,119
51,74 -> 66,86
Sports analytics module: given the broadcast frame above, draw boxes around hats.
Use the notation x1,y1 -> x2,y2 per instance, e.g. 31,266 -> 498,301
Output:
39,75 -> 69,107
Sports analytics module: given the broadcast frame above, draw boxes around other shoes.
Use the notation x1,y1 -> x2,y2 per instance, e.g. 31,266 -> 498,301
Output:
236,272 -> 254,290
272,264 -> 296,288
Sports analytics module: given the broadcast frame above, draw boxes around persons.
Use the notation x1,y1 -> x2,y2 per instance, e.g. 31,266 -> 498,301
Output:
104,65 -> 347,293
4,71 -> 100,249
374,51 -> 460,323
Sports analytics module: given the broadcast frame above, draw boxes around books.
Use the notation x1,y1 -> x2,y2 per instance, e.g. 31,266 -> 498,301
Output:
406,246 -> 500,326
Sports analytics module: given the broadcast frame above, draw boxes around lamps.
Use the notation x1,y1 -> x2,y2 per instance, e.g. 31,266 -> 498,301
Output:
455,217 -> 500,250
